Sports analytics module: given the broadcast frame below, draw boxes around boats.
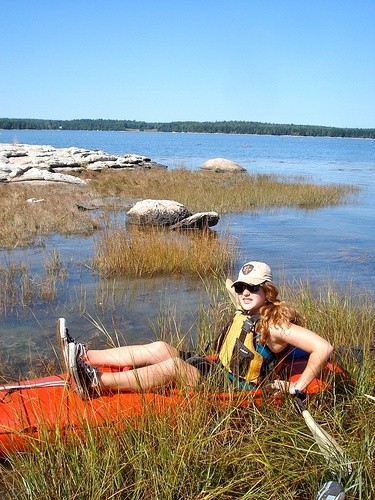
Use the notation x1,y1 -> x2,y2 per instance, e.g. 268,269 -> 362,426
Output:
1,353 -> 358,456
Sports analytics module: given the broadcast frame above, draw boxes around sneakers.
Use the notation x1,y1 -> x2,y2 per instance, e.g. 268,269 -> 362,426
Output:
56,318 -> 76,371
68,342 -> 100,400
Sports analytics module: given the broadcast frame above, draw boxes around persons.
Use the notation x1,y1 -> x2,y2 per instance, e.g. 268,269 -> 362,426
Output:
55,260 -> 335,401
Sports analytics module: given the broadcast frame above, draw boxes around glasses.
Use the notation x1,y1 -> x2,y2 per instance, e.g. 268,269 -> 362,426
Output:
234,283 -> 261,293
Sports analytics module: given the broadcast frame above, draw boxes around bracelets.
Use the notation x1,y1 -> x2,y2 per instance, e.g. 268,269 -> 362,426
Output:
294,383 -> 305,397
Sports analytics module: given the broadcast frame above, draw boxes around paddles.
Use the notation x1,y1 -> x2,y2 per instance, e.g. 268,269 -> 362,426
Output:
225,277 -> 353,480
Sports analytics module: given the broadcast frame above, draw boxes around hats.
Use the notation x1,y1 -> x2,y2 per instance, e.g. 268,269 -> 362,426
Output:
231,261 -> 273,288
